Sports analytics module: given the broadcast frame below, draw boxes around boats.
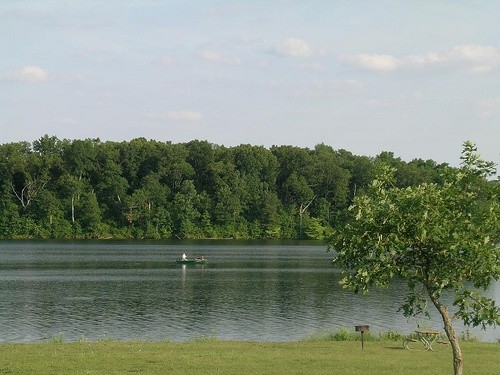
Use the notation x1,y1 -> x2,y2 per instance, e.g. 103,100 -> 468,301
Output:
176,259 -> 208,264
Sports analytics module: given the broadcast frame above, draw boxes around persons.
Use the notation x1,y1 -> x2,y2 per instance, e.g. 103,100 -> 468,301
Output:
181,253 -> 205,261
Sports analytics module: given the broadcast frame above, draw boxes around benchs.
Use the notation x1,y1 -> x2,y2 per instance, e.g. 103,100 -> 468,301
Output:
402,338 -> 418,351
437,340 -> 450,351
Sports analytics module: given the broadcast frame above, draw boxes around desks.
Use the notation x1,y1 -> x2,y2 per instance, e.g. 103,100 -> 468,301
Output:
414,330 -> 440,352
